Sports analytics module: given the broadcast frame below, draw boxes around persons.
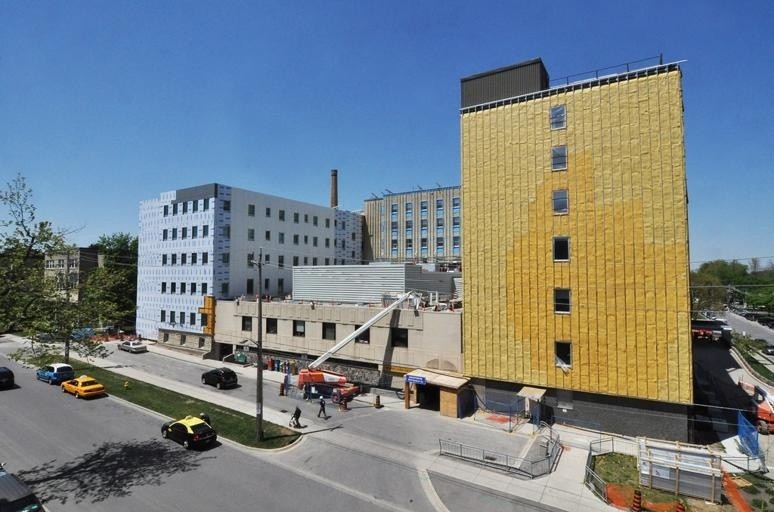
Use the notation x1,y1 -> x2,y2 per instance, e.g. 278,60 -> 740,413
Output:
316,395 -> 327,419
290,405 -> 302,429
199,412 -> 210,426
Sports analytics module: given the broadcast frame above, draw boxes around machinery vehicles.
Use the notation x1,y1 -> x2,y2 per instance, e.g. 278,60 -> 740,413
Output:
299,291 -> 412,403
736,381 -> 774,434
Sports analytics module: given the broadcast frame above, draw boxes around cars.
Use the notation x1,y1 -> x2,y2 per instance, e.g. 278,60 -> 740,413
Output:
61,375 -> 106,399
36,364 -> 76,385
763,346 -> 774,355
0,367 -> 13,391
0,462 -> 46,512
201,368 -> 237,389
118,341 -> 147,353
161,413 -> 216,451
